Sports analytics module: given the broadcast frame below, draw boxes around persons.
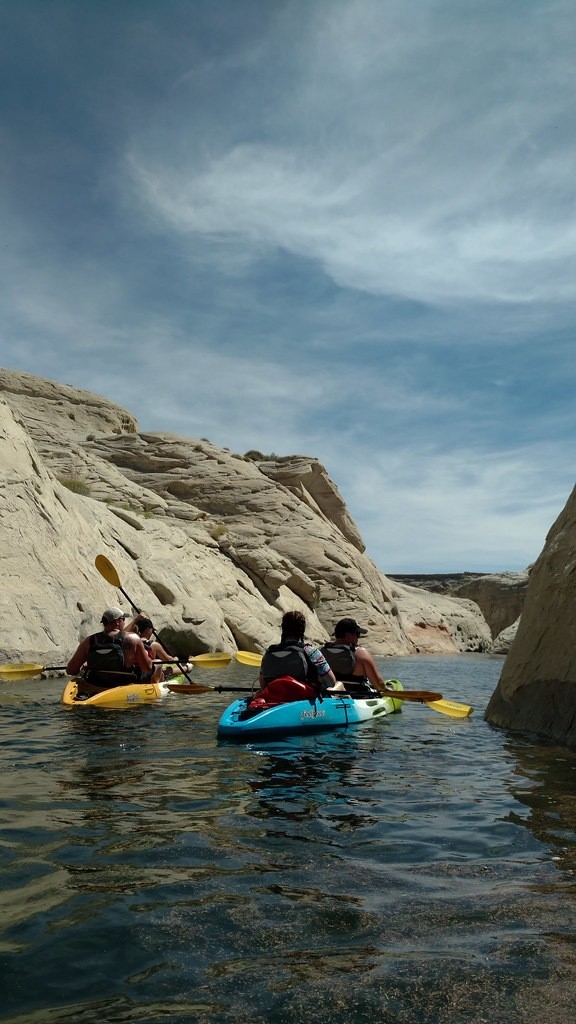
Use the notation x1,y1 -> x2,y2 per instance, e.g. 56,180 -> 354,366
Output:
66,607 -> 164,684
124,612 -> 194,678
260,611 -> 352,699
320,618 -> 393,697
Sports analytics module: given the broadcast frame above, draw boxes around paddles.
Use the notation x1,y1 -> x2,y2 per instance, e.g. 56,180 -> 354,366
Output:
236,651 -> 474,717
166,684 -> 443,703
95,554 -> 197,685
0,652 -> 232,680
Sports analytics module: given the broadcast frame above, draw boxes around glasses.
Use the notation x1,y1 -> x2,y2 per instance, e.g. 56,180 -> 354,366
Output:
119,617 -> 125,621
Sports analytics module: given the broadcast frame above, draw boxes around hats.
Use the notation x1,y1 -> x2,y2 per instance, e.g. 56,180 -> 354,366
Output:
100,606 -> 130,623
137,619 -> 157,632
331,618 -> 368,636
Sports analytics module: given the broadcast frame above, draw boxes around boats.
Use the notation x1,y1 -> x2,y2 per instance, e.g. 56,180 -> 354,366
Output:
61,665 -> 186,709
217,680 -> 403,735
